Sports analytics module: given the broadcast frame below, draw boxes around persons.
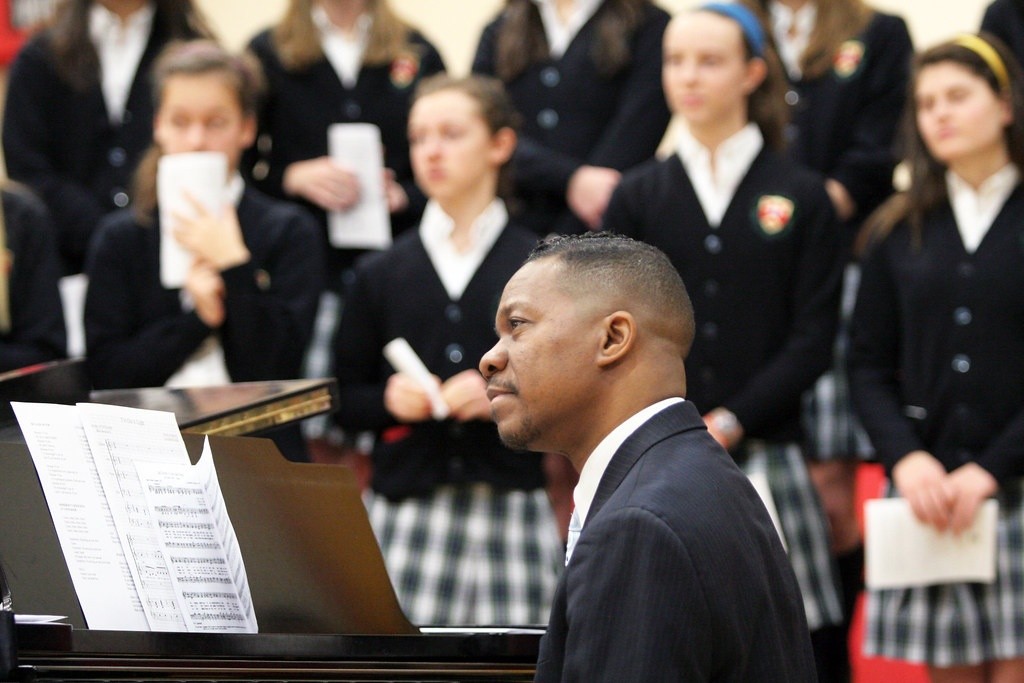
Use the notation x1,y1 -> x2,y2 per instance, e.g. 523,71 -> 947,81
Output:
331,75 -> 566,634
0,0 -> 1023,551
835,31 -> 1023,682
598,1 -> 842,645
478,230 -> 822,682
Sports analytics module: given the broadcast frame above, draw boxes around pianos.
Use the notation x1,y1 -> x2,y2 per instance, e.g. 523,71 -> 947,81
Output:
1,354 -> 547,682
